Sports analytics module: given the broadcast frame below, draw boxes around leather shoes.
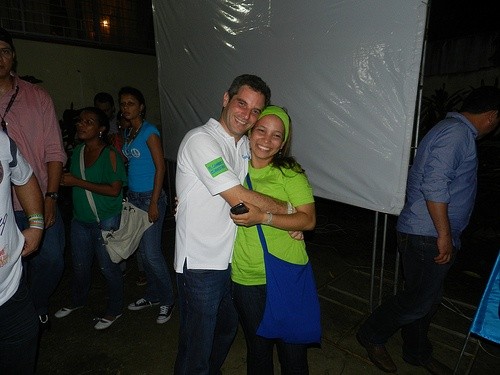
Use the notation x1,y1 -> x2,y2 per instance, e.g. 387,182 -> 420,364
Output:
355,330 -> 398,374
403,352 -> 455,375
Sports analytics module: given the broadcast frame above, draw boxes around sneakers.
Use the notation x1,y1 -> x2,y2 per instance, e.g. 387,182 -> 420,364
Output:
129,296 -> 175,325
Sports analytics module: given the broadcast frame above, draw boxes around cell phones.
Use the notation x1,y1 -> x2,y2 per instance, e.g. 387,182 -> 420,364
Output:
231,203 -> 249,215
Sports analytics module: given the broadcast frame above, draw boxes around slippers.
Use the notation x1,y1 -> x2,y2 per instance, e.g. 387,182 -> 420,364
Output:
55,305 -> 84,317
94,313 -> 124,328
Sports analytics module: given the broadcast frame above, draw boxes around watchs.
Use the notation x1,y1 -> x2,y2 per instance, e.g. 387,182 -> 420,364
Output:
45,191 -> 60,200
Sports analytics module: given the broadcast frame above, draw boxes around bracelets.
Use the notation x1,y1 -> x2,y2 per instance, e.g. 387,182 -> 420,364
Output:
28,213 -> 45,231
287,201 -> 296,215
263,211 -> 272,225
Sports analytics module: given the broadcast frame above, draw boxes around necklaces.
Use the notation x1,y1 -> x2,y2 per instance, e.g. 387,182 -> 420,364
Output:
126,123 -> 143,153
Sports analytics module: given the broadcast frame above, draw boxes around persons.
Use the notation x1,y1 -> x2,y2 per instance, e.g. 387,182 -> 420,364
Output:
173,74 -> 304,375
356,86 -> 500,375
0,26 -> 176,375
174,105 -> 316,375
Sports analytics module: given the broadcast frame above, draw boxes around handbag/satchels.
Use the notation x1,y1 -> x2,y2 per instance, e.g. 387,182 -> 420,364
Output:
257,252 -> 321,348
101,198 -> 154,264
